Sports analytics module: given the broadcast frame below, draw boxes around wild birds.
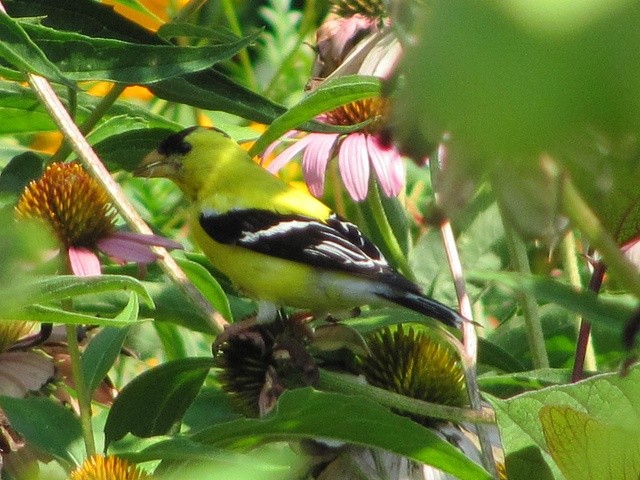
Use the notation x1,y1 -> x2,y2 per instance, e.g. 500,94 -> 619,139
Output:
132,126 -> 484,359
